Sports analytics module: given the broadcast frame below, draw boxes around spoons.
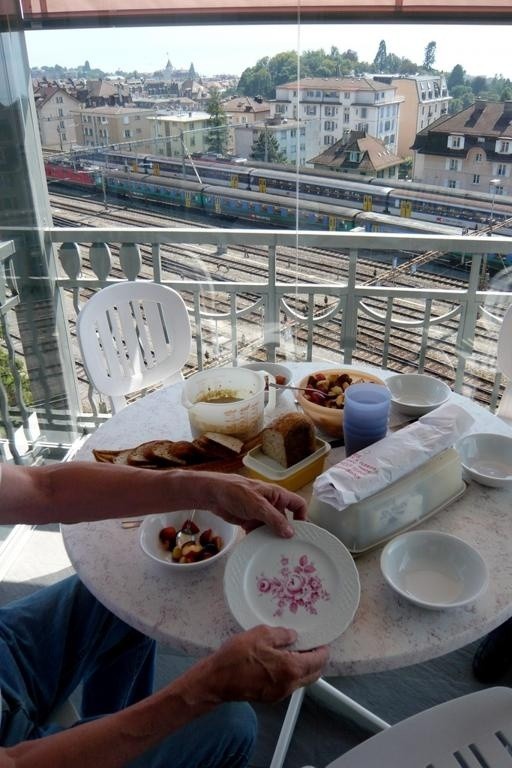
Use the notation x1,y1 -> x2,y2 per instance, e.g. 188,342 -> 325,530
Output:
269,381 -> 340,400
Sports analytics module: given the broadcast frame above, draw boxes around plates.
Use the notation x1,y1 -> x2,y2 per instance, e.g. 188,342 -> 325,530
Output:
222,519 -> 361,654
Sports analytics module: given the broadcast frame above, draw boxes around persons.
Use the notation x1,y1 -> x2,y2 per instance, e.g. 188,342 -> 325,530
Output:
0,458 -> 331,765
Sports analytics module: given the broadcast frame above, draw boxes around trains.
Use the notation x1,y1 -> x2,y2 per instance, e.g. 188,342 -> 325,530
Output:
65,147 -> 512,225
43,158 -> 512,271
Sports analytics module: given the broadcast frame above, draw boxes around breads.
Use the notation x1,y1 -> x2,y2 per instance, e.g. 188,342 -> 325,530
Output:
112,432 -> 244,468
261,411 -> 316,468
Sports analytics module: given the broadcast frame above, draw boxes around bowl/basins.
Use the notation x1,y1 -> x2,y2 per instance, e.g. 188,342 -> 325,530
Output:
384,373 -> 452,416
294,365 -> 391,439
237,361 -> 291,403
138,511 -> 238,574
378,529 -> 490,613
452,432 -> 512,490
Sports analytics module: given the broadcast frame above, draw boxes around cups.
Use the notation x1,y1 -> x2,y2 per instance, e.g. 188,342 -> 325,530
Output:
342,381 -> 393,457
182,364 -> 278,445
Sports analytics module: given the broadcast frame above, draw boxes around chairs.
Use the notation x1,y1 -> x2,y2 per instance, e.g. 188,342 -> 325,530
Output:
76,280 -> 192,417
